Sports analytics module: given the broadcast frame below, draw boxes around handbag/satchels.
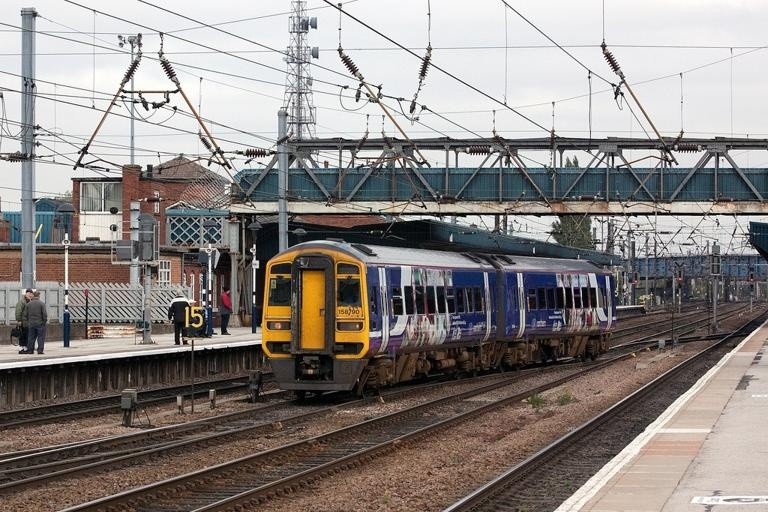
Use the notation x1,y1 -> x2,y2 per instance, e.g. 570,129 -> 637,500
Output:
11,328 -> 21,338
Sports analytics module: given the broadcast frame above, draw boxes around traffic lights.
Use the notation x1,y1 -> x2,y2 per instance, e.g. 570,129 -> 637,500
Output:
678,270 -> 682,280
634,272 -> 638,283
750,274 -> 753,282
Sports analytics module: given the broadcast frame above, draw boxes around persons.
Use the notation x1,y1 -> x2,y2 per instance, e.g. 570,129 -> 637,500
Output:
14,288 -> 34,354
167,289 -> 194,346
23,291 -> 48,354
219,286 -> 233,335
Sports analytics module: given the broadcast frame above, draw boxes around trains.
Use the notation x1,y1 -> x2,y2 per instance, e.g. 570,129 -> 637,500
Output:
262,237 -> 618,403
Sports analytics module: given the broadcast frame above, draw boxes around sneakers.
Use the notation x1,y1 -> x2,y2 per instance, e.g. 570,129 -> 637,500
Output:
173,343 -> 190,346
222,332 -> 232,335
19,349 -> 44,354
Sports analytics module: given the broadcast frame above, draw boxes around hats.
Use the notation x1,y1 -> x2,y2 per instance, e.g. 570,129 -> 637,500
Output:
176,289 -> 184,298
224,286 -> 230,292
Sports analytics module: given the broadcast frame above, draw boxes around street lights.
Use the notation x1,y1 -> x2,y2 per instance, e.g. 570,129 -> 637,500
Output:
248,222 -> 263,333
292,228 -> 307,243
55,202 -> 75,347
202,220 -> 219,336
